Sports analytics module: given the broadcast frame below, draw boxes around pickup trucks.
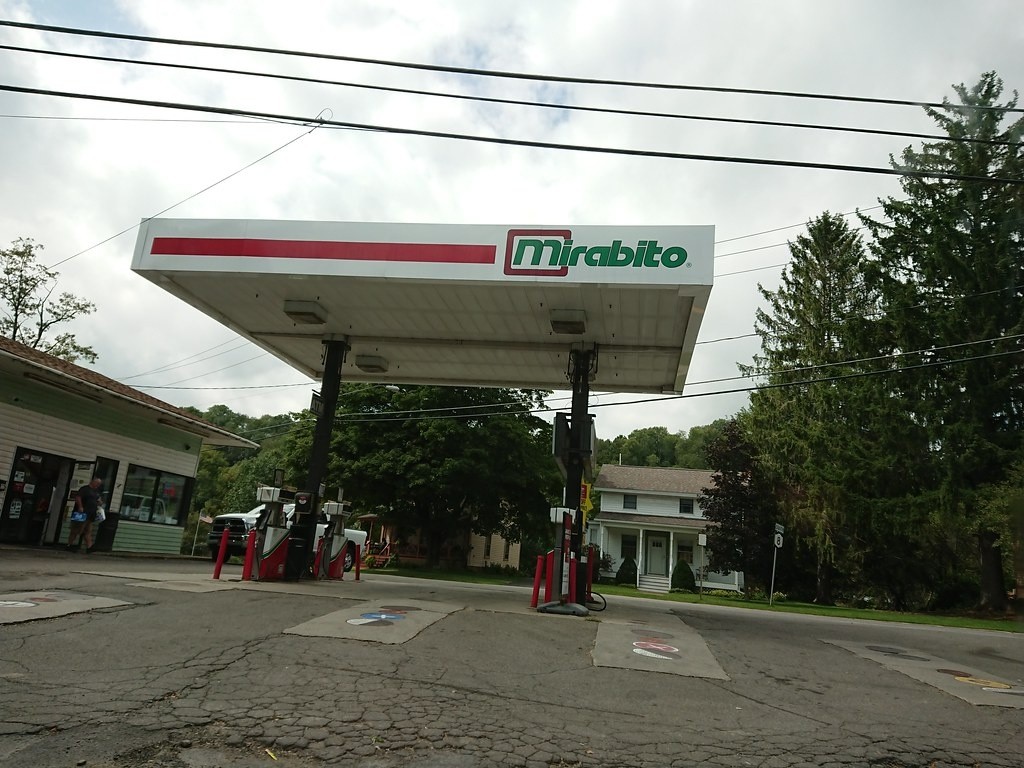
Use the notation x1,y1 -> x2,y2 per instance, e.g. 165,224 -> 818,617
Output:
208,503 -> 369,573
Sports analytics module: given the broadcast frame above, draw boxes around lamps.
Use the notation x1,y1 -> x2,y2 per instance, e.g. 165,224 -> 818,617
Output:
355,355 -> 389,373
283,299 -> 329,324
549,309 -> 586,335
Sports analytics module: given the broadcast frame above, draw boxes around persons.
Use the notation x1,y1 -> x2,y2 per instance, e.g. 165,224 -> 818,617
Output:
393,537 -> 400,544
66,477 -> 103,553
365,536 -> 373,546
35,494 -> 49,513
378,538 -> 387,553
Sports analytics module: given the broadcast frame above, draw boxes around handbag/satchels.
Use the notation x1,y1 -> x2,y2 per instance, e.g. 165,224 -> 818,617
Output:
71,511 -> 87,522
95,504 -> 106,523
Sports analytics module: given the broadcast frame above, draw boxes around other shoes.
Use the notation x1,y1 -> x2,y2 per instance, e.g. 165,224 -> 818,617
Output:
86,544 -> 97,554
66,544 -> 77,552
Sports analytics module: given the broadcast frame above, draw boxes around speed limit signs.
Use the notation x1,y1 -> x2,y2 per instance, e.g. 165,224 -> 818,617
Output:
774,531 -> 784,549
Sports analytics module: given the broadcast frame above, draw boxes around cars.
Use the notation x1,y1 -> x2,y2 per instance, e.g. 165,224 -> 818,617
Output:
101,491 -> 166,523
